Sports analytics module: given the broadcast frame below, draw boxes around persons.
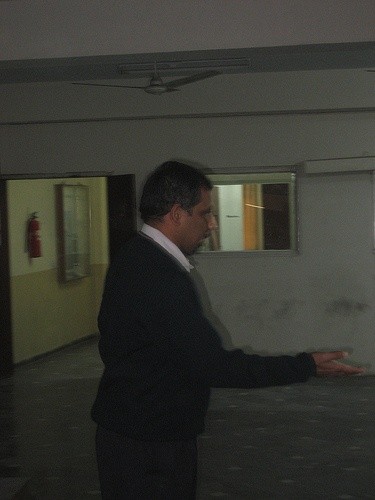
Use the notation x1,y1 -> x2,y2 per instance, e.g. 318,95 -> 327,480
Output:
87,160 -> 364,500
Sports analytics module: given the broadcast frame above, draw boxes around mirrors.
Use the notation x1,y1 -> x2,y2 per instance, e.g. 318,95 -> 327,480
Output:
188,172 -> 300,257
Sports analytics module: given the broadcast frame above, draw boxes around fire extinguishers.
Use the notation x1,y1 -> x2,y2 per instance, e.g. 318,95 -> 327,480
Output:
27,211 -> 42,258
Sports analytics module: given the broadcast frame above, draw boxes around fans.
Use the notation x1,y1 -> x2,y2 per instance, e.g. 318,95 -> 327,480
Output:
71,55 -> 222,96
